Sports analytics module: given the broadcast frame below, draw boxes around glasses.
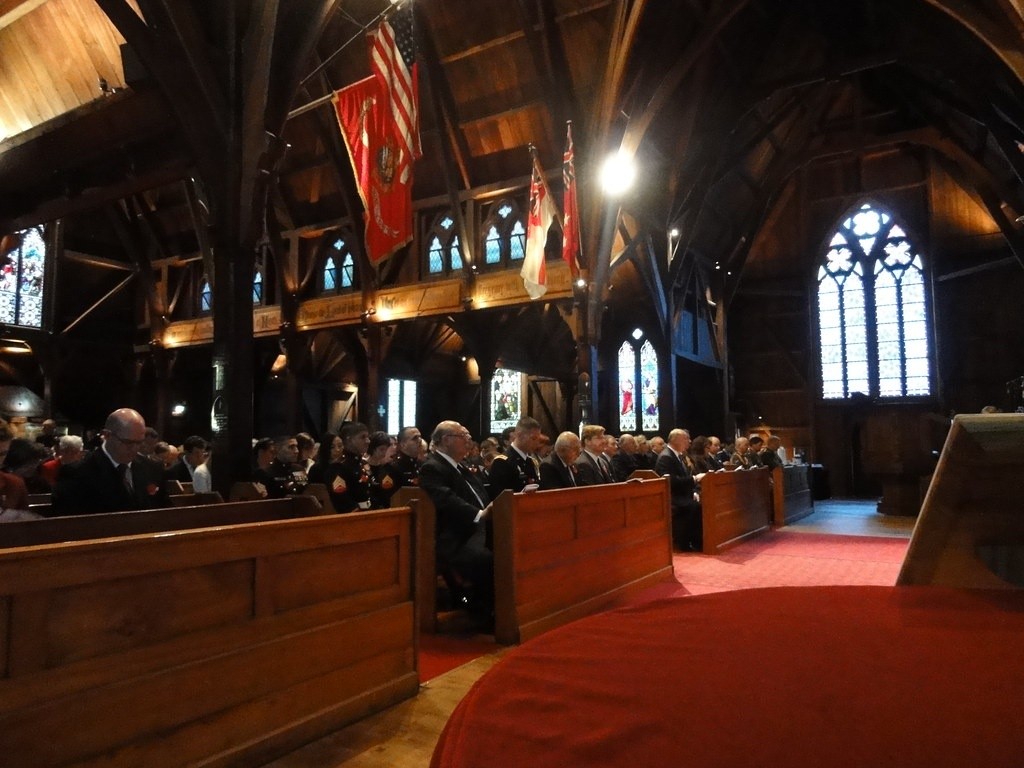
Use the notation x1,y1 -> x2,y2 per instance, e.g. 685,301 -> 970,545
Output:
446,433 -> 472,440
113,432 -> 145,448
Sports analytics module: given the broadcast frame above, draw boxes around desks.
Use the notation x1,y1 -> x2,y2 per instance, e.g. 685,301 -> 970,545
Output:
427,585 -> 1024,767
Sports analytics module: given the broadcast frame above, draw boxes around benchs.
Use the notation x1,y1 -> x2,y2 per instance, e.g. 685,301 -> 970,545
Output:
0,469 -> 662,549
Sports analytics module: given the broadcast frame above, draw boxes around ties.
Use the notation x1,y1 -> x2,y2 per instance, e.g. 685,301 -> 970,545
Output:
597,458 -> 614,483
457,463 -> 487,507
525,456 -> 537,478
116,463 -> 133,494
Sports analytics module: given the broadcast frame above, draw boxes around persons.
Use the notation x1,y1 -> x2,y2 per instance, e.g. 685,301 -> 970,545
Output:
0,407 -> 794,635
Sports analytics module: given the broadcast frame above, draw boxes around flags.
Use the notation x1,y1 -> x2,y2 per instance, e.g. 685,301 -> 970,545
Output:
521,160 -> 556,301
366,1 -> 422,162
332,74 -> 414,263
563,124 -> 583,273
